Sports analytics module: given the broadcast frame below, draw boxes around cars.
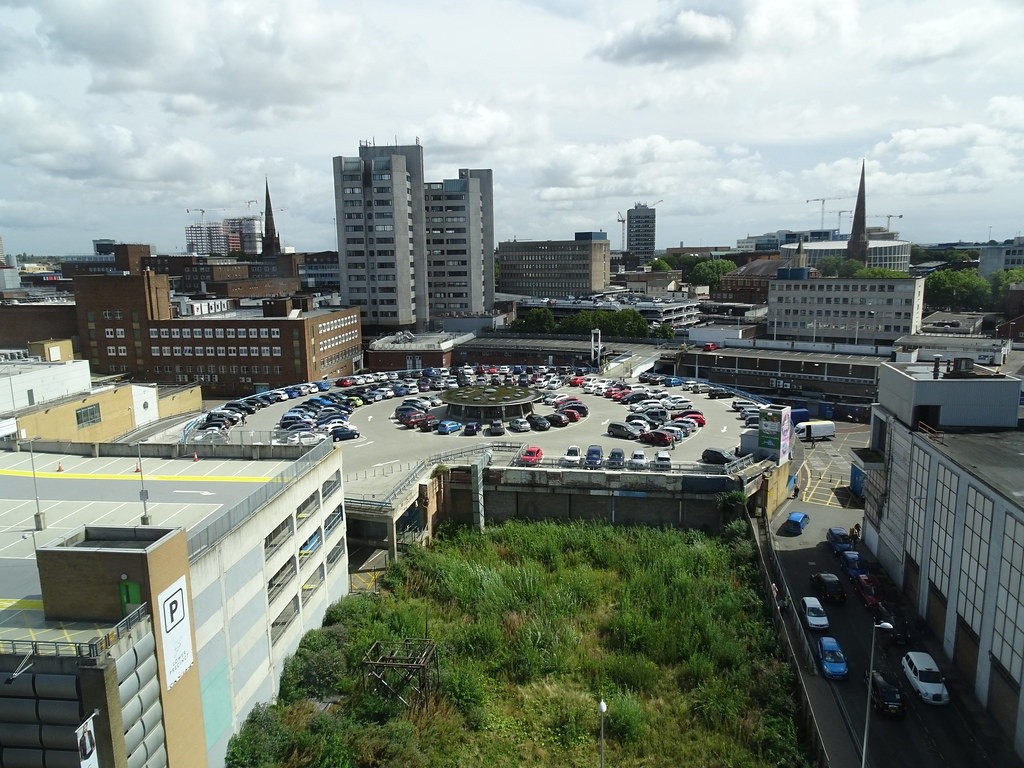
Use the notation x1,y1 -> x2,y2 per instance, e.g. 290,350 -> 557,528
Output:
809,573 -> 846,602
863,666 -> 909,719
540,295 -> 674,308
783,511 -> 811,534
871,601 -> 912,648
816,636 -> 849,682
840,551 -> 870,578
800,596 -> 831,633
853,574 -> 890,606
826,527 -> 855,554
702,342 -> 717,352
197,362 -> 772,470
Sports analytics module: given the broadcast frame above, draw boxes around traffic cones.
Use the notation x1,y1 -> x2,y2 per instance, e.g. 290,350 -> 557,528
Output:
194,451 -> 198,463
58,462 -> 63,472
135,462 -> 141,472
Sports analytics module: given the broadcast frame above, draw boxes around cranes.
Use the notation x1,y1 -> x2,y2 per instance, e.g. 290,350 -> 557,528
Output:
806,194 -> 904,233
616,211 -> 627,253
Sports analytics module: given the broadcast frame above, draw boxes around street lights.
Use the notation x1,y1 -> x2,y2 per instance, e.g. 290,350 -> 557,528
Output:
17,436 -> 47,532
598,698 -> 608,768
860,623 -> 896,768
127,437 -> 150,526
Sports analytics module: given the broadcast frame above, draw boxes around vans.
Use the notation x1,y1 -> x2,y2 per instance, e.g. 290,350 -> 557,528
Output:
793,420 -> 836,442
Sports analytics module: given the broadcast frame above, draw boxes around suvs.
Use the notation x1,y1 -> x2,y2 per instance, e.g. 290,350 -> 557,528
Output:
900,651 -> 951,707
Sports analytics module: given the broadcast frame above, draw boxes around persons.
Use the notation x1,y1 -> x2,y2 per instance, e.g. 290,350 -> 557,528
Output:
679,430 -> 683,442
793,485 -> 799,498
671,437 -> 675,450
812,437 -> 815,448
241,414 -> 247,426
854,523 -> 861,537
